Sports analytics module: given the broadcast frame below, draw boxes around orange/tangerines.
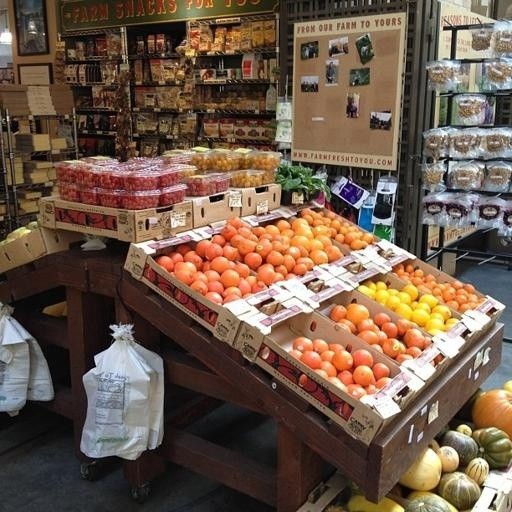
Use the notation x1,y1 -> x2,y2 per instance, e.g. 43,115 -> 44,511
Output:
288,261 -> 486,399
144,208 -> 376,325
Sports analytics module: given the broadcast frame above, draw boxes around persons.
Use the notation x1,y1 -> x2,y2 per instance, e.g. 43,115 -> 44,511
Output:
326,62 -> 337,83
307,43 -> 315,59
301,81 -> 318,92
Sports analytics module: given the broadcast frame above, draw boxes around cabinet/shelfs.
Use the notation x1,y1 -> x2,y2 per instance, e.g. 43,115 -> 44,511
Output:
54,0 -> 286,166
1,107 -> 79,238
115,272 -> 506,512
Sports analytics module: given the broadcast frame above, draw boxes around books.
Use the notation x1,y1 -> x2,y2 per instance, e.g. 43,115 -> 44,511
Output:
0,81 -> 74,221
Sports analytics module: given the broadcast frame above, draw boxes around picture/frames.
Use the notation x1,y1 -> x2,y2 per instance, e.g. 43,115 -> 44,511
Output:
11,0 -> 50,57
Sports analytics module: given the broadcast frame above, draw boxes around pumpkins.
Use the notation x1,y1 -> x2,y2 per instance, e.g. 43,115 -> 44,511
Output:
348,381 -> 512,512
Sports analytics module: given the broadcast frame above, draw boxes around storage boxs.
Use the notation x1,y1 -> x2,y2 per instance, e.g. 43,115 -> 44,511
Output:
47,181 -> 284,242
295,456 -> 512,511
40,184 -> 509,446
1,227 -> 87,274
